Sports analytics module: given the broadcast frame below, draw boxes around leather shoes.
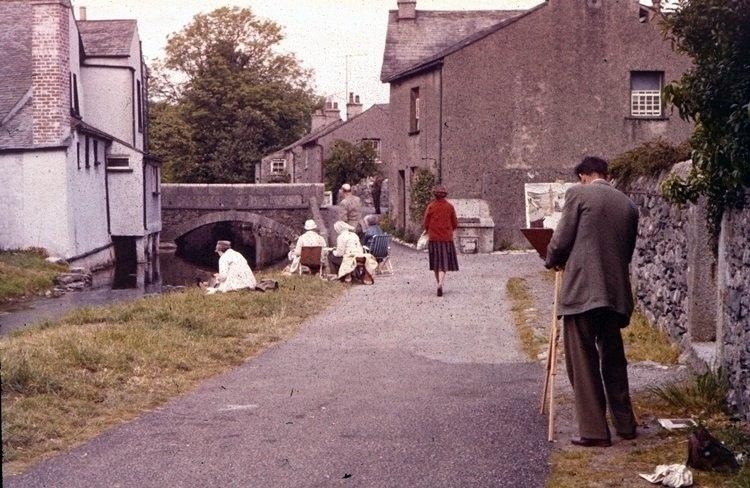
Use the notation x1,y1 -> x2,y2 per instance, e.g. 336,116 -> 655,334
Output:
571,437 -> 612,447
437,286 -> 443,296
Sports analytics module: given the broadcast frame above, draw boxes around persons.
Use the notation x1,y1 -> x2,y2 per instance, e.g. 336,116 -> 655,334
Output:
337,184 -> 368,247
370,175 -> 381,214
543,156 -> 639,448
361,214 -> 387,264
197,241 -> 257,295
327,221 -> 363,274
287,219 -> 327,276
422,184 -> 459,297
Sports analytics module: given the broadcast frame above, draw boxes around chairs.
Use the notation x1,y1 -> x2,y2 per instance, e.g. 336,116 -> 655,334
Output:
337,253 -> 376,284
299,246 -> 323,278
363,235 -> 391,275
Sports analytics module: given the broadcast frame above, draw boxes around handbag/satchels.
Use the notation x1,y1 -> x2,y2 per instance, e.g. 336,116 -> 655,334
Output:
416,229 -> 429,251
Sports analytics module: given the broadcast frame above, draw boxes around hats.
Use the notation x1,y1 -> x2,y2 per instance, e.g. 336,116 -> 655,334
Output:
214,240 -> 231,252
304,219 -> 317,230
